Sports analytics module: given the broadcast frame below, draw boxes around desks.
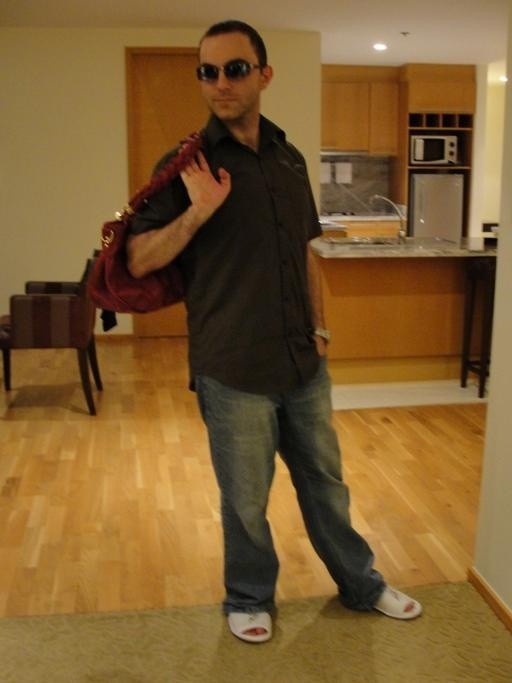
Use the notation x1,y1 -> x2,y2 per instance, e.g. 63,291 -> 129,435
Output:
460,257 -> 495,396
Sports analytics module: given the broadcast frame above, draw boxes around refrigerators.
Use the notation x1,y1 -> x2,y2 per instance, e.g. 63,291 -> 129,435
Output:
408,171 -> 464,237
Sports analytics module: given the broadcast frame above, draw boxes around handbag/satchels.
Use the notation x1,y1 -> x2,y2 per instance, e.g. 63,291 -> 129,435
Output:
83,129 -> 209,312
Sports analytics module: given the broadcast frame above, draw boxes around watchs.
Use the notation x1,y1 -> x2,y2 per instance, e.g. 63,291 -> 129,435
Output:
313,328 -> 333,342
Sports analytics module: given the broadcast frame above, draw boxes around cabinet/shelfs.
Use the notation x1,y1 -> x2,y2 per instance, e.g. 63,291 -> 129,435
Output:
320,66 -> 369,151
369,65 -> 399,153
389,65 -> 476,237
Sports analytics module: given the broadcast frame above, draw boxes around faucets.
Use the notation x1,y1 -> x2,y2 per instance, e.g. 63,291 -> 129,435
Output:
368,193 -> 407,246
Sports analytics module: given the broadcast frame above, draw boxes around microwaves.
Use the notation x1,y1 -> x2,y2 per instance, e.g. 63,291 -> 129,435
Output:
409,134 -> 461,166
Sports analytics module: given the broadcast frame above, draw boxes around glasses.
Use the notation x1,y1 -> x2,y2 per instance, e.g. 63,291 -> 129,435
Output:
196,58 -> 266,81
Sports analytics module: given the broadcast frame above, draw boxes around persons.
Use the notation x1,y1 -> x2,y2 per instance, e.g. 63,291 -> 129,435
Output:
123,20 -> 423,644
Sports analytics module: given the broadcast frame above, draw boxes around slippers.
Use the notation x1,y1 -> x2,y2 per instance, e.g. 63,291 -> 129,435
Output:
227,610 -> 274,643
373,583 -> 423,621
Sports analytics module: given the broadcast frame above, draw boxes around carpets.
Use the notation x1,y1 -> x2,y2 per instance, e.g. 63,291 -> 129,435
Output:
0,579 -> 512,683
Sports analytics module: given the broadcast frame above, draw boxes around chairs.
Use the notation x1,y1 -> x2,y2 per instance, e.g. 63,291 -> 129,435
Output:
0,249 -> 103,415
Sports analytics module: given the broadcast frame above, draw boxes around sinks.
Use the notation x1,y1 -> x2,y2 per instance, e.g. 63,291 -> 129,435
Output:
328,236 -> 400,245
